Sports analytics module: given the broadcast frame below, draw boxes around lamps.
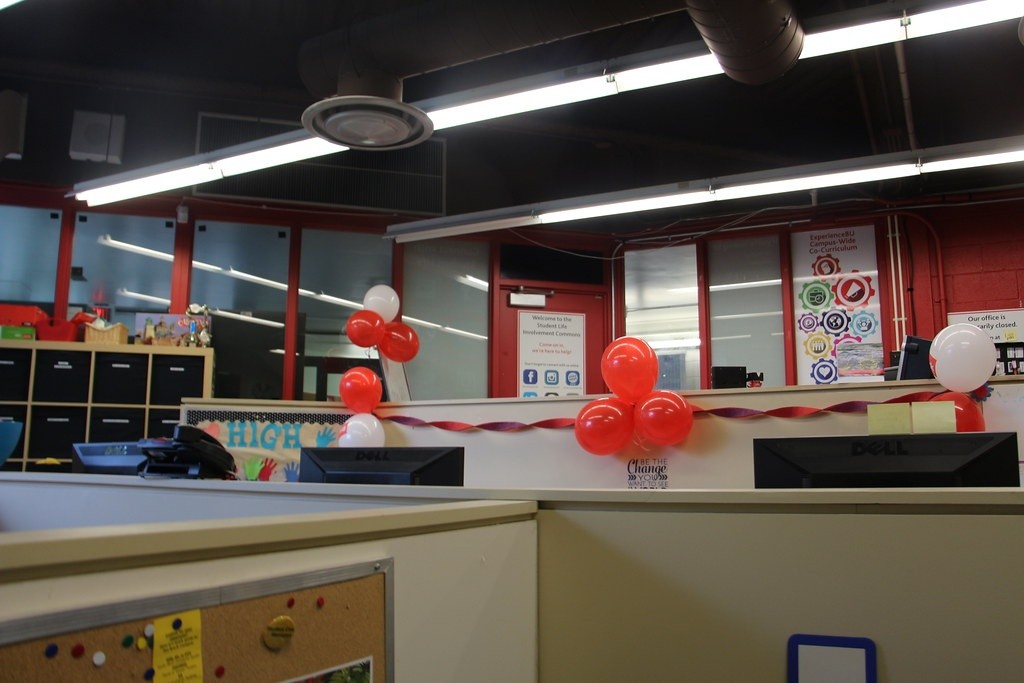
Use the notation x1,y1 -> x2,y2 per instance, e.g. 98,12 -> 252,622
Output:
66,0 -> 1024,240
99,241 -> 489,342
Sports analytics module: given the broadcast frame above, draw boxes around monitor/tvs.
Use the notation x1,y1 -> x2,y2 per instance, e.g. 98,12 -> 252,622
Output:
301,447 -> 465,487
70,442 -> 149,476
752,431 -> 1021,488
896,335 -> 932,379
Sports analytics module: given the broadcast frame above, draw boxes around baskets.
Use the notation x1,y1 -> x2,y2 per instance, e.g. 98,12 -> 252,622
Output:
84,322 -> 129,345
36,318 -> 78,341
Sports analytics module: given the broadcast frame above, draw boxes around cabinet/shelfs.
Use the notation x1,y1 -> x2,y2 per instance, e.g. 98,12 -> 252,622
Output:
0,338 -> 214,471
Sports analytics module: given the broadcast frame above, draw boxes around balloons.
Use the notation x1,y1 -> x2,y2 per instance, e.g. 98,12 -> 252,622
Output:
347,310 -> 386,347
340,367 -> 383,413
575,397 -> 635,454
363,285 -> 399,323
601,336 -> 659,403
339,413 -> 386,447
930,392 -> 985,432
929,323 -> 998,392
635,390 -> 693,445
379,321 -> 419,362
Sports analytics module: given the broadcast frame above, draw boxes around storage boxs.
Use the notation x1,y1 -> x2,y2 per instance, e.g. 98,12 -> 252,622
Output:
0,326 -> 35,340
134,312 -> 211,343
